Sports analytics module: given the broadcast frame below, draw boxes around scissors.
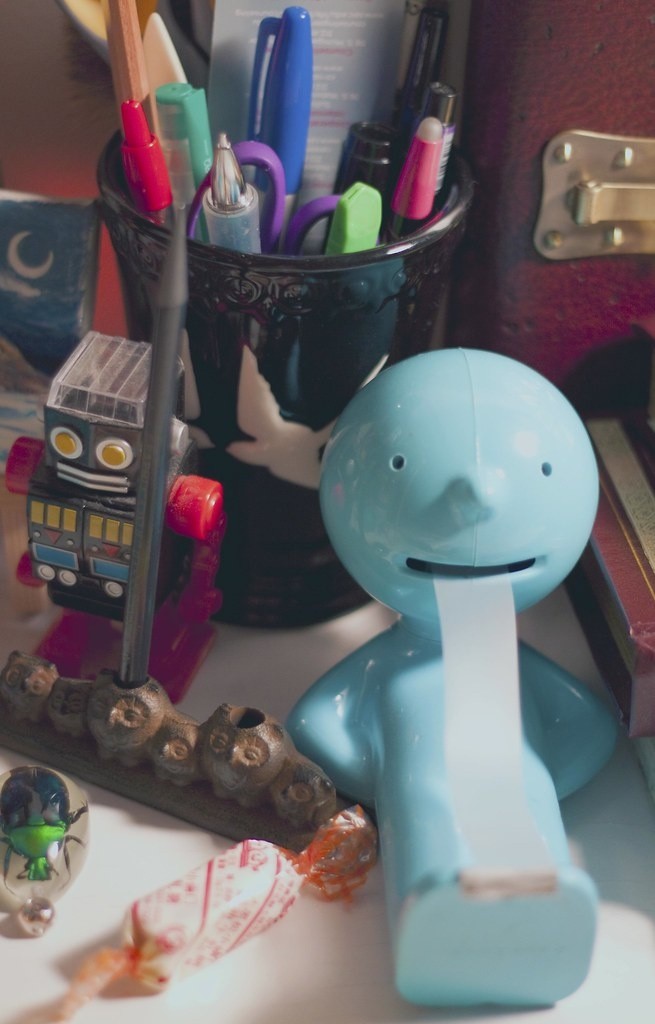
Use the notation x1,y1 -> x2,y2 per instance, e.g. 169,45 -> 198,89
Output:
175,133 -> 350,260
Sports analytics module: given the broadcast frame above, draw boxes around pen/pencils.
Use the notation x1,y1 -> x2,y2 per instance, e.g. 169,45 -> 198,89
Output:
114,8 -> 460,264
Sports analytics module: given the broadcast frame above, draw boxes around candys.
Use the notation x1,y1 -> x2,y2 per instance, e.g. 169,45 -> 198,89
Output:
44,802 -> 380,1020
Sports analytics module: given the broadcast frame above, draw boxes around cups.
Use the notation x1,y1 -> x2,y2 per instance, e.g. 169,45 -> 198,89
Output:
95,128 -> 480,631
